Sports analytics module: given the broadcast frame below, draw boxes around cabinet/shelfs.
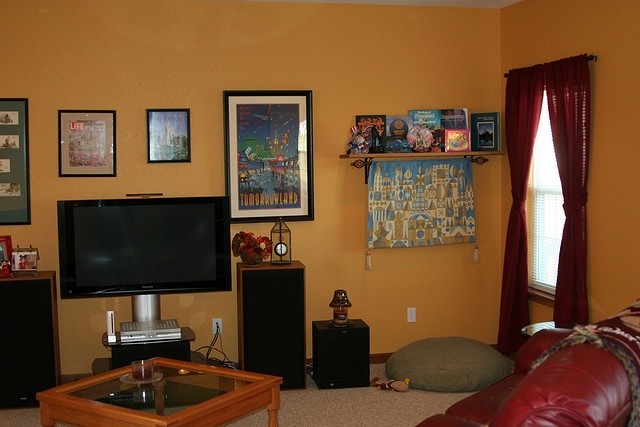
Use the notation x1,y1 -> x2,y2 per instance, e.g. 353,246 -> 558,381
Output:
91,326 -> 212,372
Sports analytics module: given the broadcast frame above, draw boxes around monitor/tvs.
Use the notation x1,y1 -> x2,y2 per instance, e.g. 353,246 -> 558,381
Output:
57,193 -> 232,347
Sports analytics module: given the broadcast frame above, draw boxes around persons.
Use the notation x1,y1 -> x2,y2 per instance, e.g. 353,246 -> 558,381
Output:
18,255 -> 29,269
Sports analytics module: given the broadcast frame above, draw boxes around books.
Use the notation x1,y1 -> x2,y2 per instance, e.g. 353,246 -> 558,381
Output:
408,110 -> 441,152
441,108 -> 468,152
386,116 -> 412,153
355,115 -> 386,153
444,128 -> 471,152
471,112 -> 499,150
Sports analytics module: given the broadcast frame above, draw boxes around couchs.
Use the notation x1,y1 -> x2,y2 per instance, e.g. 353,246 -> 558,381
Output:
408,301 -> 639,426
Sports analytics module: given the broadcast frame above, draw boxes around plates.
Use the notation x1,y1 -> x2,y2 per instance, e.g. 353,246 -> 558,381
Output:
120,372 -> 163,385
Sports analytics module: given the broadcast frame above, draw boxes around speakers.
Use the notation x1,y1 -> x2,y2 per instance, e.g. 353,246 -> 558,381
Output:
238,260 -> 306,392
312,318 -> 370,388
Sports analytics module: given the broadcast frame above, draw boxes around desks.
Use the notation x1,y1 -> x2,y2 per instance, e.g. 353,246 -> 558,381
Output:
35,356 -> 285,426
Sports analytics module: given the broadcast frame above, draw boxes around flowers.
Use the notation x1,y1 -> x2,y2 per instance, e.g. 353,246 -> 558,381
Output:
232,231 -> 272,260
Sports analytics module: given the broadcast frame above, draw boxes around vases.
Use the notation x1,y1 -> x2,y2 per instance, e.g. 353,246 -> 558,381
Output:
240,254 -> 261,267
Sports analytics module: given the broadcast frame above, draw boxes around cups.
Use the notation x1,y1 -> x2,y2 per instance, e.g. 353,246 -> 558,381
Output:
132,360 -> 155,380
132,387 -> 155,404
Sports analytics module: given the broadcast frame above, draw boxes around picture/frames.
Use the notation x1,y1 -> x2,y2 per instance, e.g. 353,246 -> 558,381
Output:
11,251 -> 37,270
0,235 -> 14,279
146,107 -> 192,165
56,108 -> 116,179
223,89 -> 314,223
0,97 -> 33,225
